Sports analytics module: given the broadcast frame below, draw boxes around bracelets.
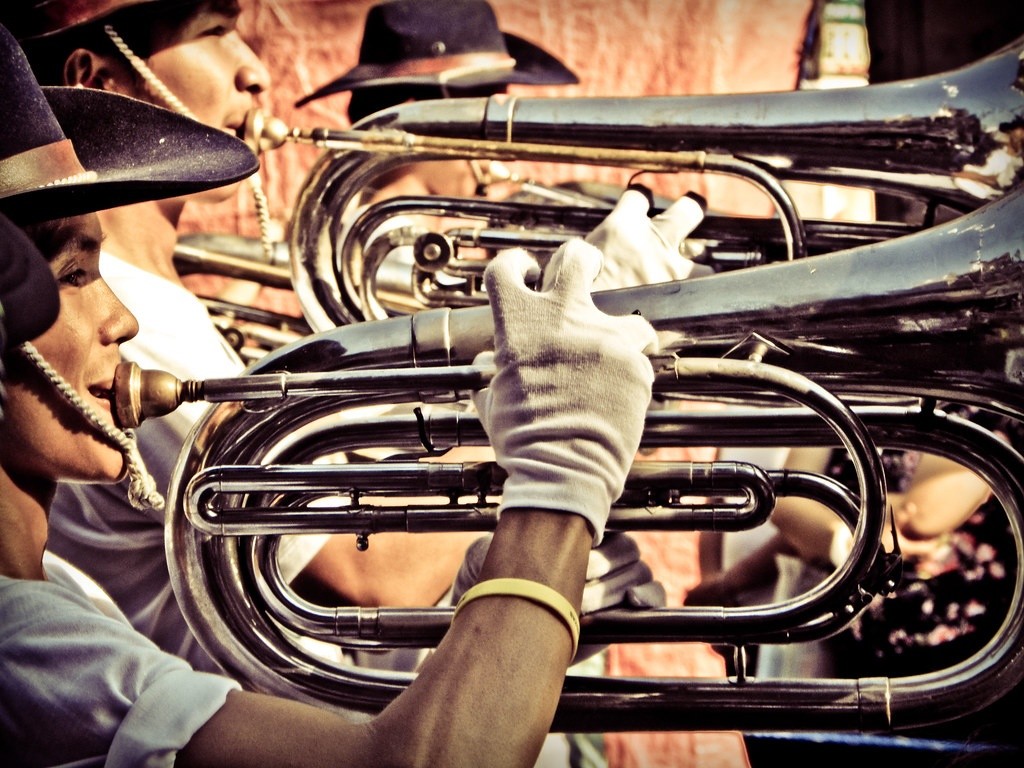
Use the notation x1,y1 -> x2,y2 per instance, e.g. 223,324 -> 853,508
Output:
451,574 -> 583,661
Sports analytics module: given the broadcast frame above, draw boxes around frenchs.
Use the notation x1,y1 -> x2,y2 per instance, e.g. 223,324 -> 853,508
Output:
109,187 -> 1024,734
240,34 -> 1024,342
167,158 -> 712,365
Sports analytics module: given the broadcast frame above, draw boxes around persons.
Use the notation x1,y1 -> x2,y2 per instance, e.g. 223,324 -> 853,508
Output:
0,0 -> 1022,767
345,90 -> 504,245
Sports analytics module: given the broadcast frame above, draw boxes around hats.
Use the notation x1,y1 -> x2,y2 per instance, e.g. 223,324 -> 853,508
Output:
1,11 -> 260,221
0,0 -> 140,41
294,1 -> 579,107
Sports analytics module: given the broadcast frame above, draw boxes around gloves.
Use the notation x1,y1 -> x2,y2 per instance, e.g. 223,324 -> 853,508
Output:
562,191 -> 706,353
452,522 -> 666,664
470,237 -> 659,546
